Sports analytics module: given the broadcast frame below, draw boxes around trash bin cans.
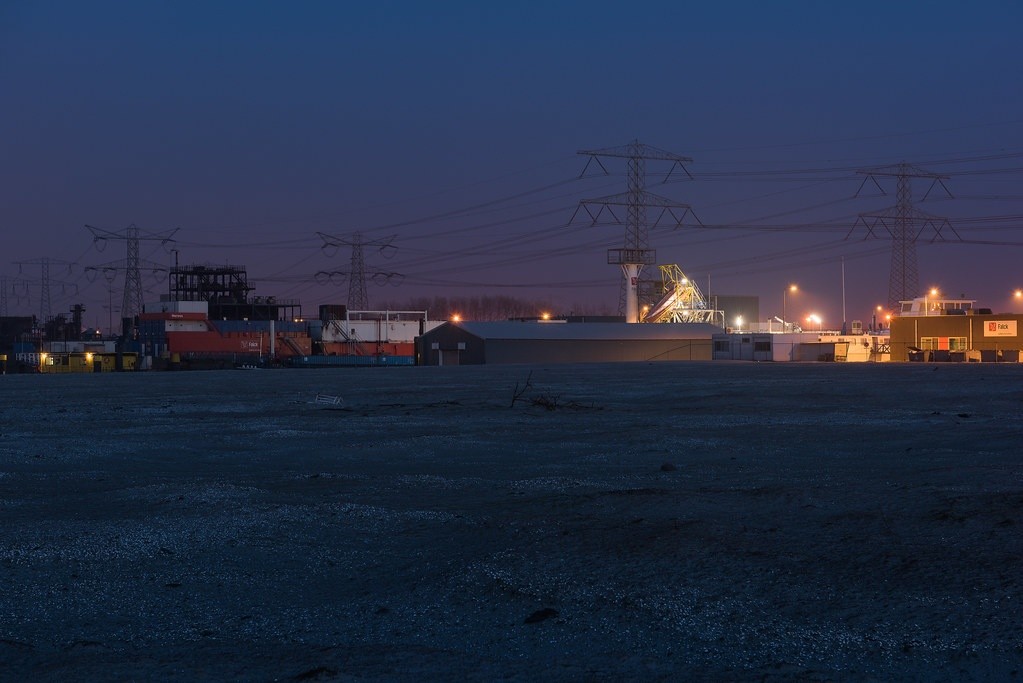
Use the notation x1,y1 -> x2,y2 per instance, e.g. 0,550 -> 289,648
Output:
319,304 -> 347,320
934,350 -> 967,362
980,349 -> 1019,363
907,346 -> 924,362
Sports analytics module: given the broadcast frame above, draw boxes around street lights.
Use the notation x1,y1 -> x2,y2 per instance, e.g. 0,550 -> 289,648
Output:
925,288 -> 937,316
782,285 -> 795,331
